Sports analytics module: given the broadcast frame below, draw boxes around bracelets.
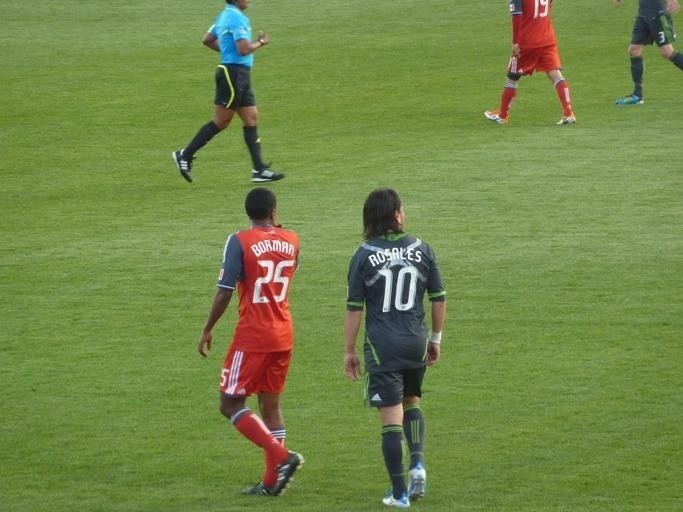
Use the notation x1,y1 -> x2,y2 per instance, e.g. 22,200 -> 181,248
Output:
429,332 -> 441,344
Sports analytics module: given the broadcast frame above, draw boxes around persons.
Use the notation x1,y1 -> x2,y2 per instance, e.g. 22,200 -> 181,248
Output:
484,0 -> 577,125
172,0 -> 286,183
613,0 -> 683,106
197,187 -> 306,497
343,187 -> 447,508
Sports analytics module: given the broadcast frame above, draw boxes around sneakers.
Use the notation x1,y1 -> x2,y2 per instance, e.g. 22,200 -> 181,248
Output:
382,462 -> 425,509
242,450 -> 304,496
484,110 -> 509,125
173,149 -> 193,183
615,94 -> 644,104
556,111 -> 576,125
251,168 -> 285,182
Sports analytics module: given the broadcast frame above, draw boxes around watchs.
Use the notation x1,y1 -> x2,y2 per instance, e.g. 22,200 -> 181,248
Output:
258,38 -> 265,46
